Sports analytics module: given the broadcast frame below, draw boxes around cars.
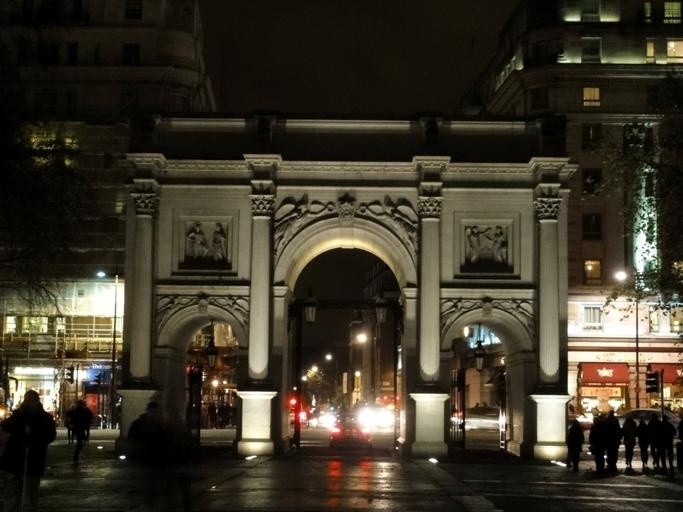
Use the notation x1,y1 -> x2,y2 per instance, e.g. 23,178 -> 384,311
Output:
328,414 -> 372,449
463,406 -> 502,432
582,408 -> 680,446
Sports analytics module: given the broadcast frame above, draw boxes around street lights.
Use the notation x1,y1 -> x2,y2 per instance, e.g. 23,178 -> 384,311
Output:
354,371 -> 361,404
347,320 -> 366,412
96,270 -> 118,430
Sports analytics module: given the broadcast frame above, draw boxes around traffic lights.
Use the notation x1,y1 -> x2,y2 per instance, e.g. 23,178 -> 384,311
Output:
645,373 -> 657,393
289,398 -> 297,407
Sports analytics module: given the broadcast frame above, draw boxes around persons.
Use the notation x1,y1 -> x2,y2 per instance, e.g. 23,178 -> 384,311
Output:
212,222 -> 226,261
466,226 -> 490,265
185,223 -> 209,258
0,388 -> 57,511
125,400 -> 160,512
588,408 -> 683,474
565,420 -> 585,470
70,399 -> 85,448
64,405 -> 76,445
485,225 -> 507,263
217,402 -> 227,429
81,406 -> 90,443
200,401 -> 209,429
206,401 -> 219,429
